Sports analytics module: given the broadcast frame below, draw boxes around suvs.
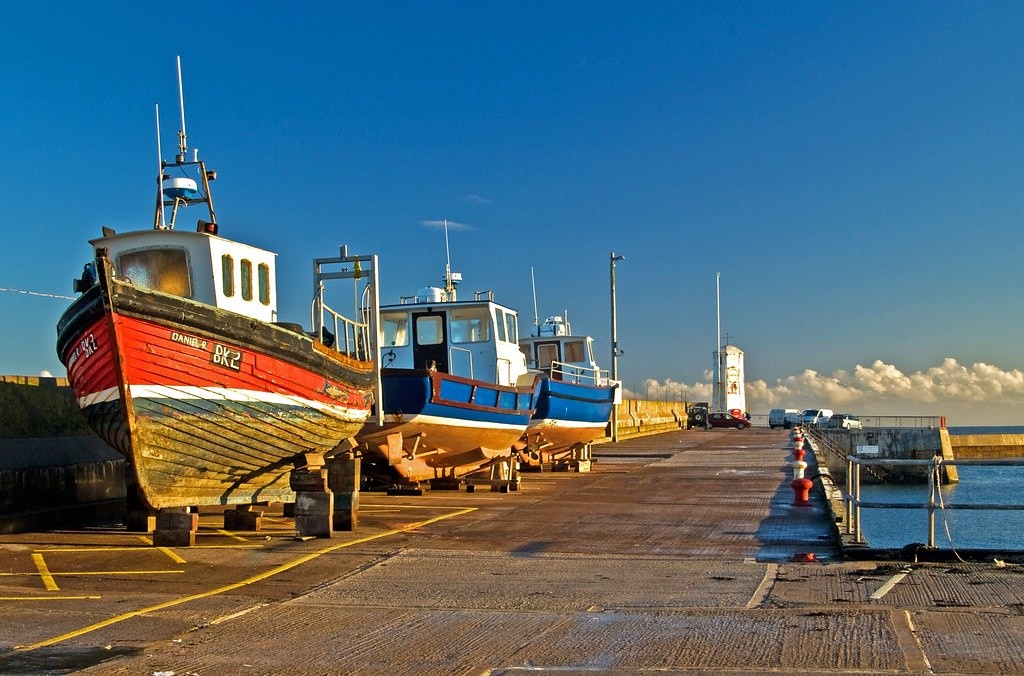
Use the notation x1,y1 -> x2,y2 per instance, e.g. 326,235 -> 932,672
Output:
685,406 -> 710,431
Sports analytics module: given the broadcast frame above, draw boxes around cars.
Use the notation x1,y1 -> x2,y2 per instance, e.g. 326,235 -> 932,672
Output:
706,411 -> 752,430
828,413 -> 863,431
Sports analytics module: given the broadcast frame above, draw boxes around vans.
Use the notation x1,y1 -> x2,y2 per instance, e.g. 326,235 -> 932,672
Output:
798,408 -> 834,429
769,408 -> 804,429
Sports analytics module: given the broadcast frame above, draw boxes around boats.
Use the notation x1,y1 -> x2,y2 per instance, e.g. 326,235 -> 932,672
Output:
341,217 -> 545,484
518,264 -> 620,475
57,54 -> 383,514
